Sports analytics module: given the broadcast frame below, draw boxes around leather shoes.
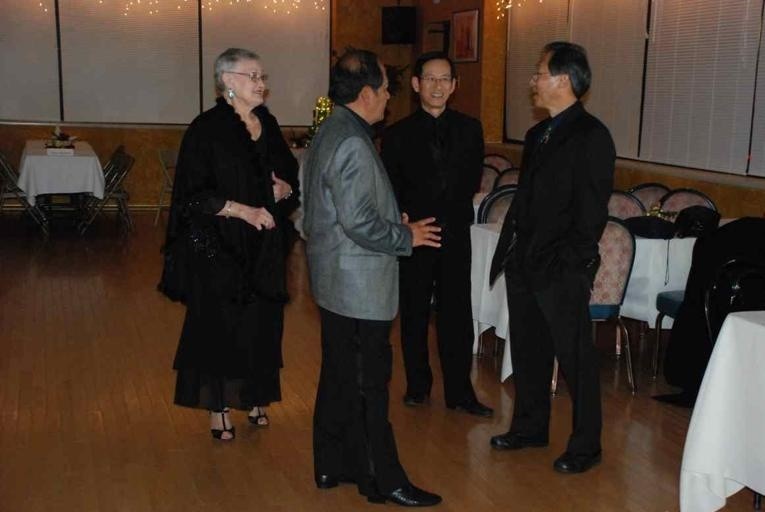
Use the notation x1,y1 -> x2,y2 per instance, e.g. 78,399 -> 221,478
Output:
446,397 -> 495,417
315,473 -> 361,487
554,448 -> 601,474
368,480 -> 443,506
403,384 -> 431,403
491,431 -> 548,450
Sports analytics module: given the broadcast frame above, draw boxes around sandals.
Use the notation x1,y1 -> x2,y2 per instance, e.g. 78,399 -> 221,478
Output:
209,406 -> 235,441
248,405 -> 268,427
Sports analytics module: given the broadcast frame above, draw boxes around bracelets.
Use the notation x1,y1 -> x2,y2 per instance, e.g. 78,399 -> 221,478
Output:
226,200 -> 234,216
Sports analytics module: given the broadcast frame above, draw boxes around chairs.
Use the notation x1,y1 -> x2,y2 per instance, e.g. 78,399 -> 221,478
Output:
480,165 -> 499,193
651,216 -> 764,408
152,148 -> 175,228
548,216 -> 635,396
64,145 -> 134,238
476,184 -> 517,223
659,189 -> 717,215
469,222 -> 697,384
493,169 -> 519,189
483,153 -> 512,171
628,181 -> 670,215
0,152 -> 62,236
608,190 -> 646,216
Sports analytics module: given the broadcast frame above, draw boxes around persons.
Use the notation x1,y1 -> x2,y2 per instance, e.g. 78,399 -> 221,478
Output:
488,39 -> 616,476
300,46 -> 443,508
155,45 -> 299,442
380,51 -> 496,418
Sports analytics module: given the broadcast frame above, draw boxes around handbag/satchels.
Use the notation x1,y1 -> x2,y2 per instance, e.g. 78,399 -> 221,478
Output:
666,205 -> 722,285
624,214 -> 675,240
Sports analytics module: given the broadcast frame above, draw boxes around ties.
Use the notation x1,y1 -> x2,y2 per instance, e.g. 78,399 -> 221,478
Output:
431,120 -> 446,189
531,122 -> 552,174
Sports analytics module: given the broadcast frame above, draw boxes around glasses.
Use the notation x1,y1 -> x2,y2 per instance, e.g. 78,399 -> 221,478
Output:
529,71 -> 554,80
222,70 -> 268,83
418,75 -> 452,83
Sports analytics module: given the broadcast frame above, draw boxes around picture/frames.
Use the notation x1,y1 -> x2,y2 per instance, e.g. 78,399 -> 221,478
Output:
449,8 -> 479,63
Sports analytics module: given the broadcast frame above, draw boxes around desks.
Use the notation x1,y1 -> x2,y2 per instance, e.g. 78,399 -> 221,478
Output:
17,138 -> 105,242
678,310 -> 765,510
